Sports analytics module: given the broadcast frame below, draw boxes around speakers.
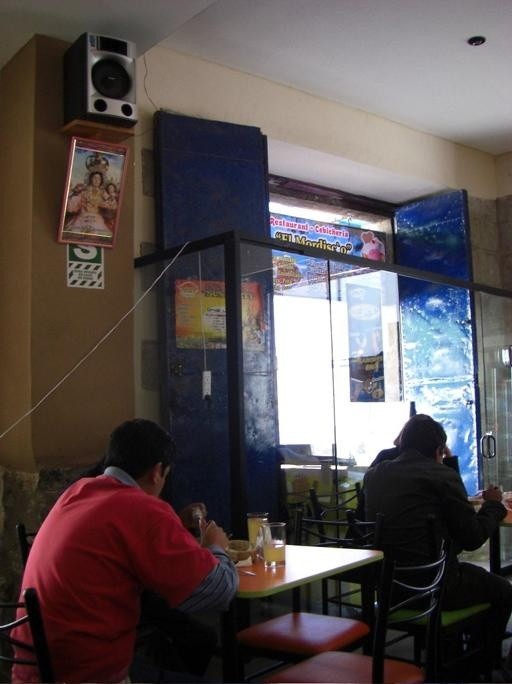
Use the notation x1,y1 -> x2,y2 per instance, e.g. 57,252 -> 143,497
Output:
62,32 -> 140,125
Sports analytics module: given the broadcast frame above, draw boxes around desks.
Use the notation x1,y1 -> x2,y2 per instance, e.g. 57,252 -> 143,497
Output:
144,538 -> 380,680
470,494 -> 511,578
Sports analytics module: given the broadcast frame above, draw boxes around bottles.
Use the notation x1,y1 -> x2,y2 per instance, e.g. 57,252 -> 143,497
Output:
190,504 -> 207,546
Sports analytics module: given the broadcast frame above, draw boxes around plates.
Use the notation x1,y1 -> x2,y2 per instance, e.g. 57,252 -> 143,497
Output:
467,496 -> 488,506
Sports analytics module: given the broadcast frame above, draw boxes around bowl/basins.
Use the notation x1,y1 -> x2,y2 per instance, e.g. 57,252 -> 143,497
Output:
229,540 -> 255,562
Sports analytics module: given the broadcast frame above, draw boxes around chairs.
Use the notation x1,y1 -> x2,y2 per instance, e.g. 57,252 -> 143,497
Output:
1,589 -> 50,684
311,483 -> 491,682
227,519 -> 380,670
271,539 -> 445,684
309,485 -> 369,619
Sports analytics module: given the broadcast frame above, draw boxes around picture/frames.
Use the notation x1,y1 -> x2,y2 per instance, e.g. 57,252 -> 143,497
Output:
56,134 -> 131,249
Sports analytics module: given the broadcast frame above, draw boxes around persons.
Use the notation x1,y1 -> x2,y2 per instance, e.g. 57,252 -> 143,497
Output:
8,418 -> 240,684
39,446 -> 172,525
359,418 -> 511,683
341,414 -> 459,547
64,171 -> 118,240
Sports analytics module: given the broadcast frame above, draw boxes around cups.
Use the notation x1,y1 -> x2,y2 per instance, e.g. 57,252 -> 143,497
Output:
244,511 -> 286,573
